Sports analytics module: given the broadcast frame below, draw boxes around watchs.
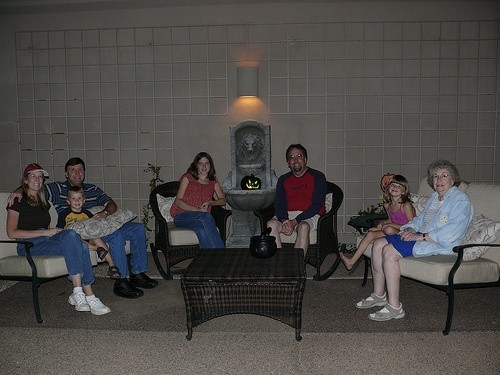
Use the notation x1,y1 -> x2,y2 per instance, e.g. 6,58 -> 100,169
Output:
103,209 -> 111,215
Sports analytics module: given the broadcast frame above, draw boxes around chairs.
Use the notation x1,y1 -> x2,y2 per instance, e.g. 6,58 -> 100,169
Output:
149,181 -> 232,280
254,181 -> 346,281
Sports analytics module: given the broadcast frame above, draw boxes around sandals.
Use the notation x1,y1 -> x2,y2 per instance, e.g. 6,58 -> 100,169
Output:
108,266 -> 122,278
355,292 -> 387,309
367,302 -> 405,321
96,246 -> 108,260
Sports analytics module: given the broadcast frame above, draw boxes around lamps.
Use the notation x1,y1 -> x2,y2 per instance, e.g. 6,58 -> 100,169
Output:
237,67 -> 259,97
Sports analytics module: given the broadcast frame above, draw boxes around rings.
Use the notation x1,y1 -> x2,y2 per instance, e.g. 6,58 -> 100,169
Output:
8,197 -> 10,199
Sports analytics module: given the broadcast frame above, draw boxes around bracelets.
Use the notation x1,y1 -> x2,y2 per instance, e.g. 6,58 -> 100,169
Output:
422,233 -> 426,241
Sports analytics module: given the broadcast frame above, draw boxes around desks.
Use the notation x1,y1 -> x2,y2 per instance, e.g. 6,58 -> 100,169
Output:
181,248 -> 306,343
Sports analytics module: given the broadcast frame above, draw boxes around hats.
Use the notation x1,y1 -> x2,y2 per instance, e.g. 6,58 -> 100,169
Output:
26,163 -> 49,177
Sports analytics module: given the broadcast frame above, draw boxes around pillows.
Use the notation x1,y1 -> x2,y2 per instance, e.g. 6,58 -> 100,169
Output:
156,194 -> 176,223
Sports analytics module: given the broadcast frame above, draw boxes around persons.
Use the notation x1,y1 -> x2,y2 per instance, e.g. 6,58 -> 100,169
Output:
356,160 -> 474,322
170,152 -> 226,248
339,175 -> 416,270
57,186 -> 121,279
267,144 -> 326,259
6,163 -> 111,315
6,157 -> 158,298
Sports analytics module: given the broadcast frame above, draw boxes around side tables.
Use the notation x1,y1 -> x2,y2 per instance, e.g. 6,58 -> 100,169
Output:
348,214 -> 386,235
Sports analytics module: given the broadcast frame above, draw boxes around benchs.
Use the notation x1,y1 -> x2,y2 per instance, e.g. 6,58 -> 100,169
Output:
0,193 -> 131,323
355,176 -> 500,336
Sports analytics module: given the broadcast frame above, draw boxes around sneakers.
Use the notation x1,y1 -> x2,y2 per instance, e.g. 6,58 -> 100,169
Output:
113,277 -> 144,298
68,292 -> 90,311
87,297 -> 111,316
130,272 -> 158,289
